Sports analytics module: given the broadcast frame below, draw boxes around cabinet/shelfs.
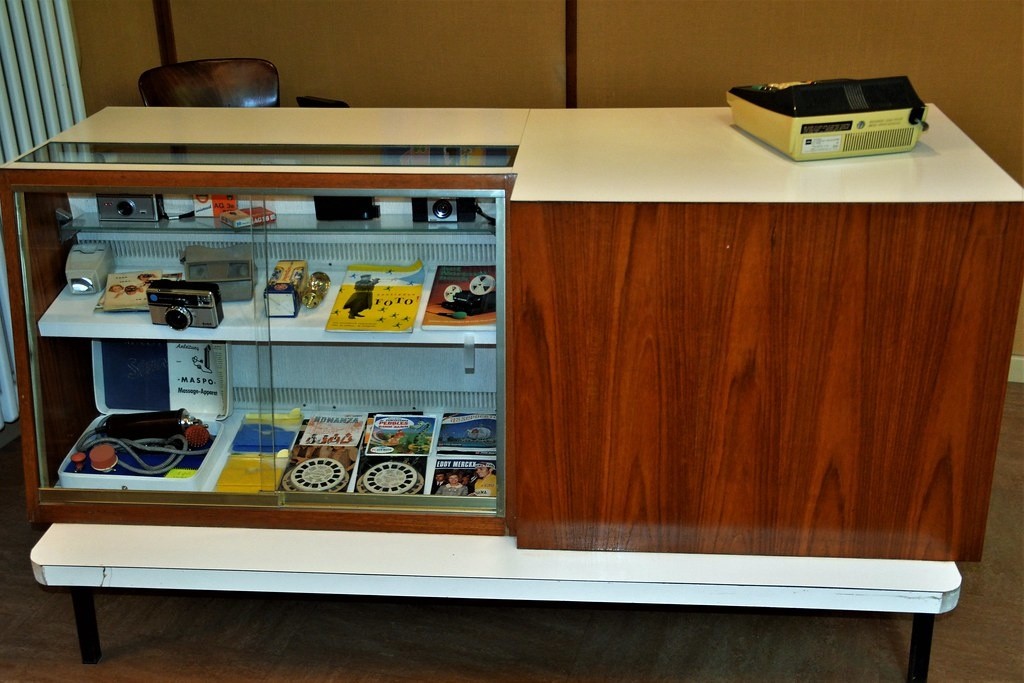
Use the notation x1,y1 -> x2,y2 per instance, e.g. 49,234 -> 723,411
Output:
0,102 -> 1024,683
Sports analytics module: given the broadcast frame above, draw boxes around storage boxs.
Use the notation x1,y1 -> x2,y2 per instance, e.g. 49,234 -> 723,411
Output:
264,259 -> 309,318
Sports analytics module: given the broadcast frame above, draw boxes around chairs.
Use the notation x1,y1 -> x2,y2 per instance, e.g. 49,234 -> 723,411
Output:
139,58 -> 280,107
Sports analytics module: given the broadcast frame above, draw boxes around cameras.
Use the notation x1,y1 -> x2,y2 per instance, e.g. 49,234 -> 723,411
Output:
96,193 -> 164,222
147,280 -> 225,329
313,195 -> 375,221
413,196 -> 477,223
